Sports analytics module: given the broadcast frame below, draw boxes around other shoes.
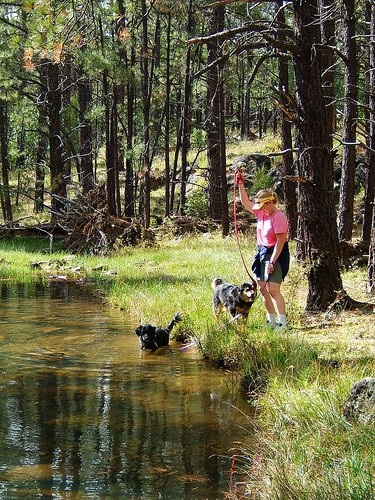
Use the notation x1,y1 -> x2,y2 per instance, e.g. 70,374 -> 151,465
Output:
267,321 -> 275,327
274,324 -> 287,331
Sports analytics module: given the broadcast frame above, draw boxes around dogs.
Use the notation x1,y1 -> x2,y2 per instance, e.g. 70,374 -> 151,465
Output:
135,310 -> 183,353
210,277 -> 258,335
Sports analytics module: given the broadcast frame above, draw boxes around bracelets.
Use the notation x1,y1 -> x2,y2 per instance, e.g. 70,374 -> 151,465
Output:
269,261 -> 275,267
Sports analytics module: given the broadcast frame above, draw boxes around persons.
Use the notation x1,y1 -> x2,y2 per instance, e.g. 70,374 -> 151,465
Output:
235,167 -> 290,328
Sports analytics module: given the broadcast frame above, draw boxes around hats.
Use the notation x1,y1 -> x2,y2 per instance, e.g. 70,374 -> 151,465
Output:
252,195 -> 275,210
